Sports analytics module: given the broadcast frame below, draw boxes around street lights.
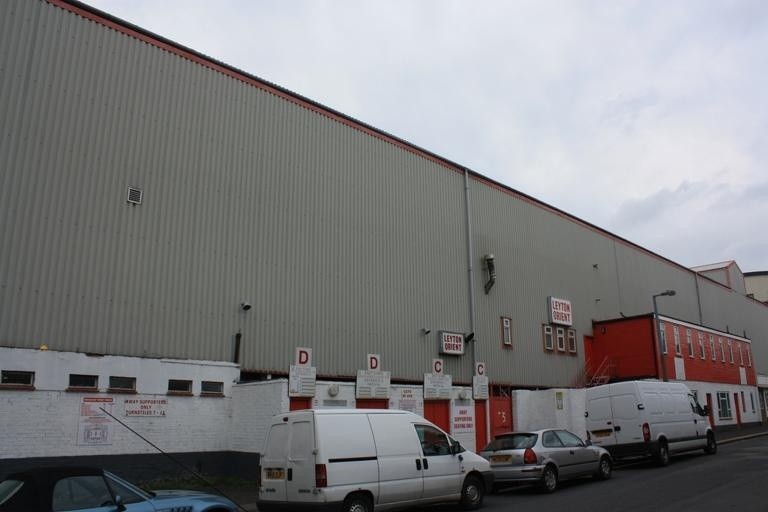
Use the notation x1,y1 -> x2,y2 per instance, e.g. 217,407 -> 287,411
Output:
653,285 -> 676,380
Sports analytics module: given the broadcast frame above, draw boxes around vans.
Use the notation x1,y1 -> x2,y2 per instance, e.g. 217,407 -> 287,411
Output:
582,380 -> 717,465
254,407 -> 492,512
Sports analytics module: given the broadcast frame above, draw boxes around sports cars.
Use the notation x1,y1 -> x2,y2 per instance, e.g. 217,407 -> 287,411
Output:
0,460 -> 241,512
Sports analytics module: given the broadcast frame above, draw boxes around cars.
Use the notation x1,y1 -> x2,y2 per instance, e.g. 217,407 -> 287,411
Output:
481,427 -> 613,491
